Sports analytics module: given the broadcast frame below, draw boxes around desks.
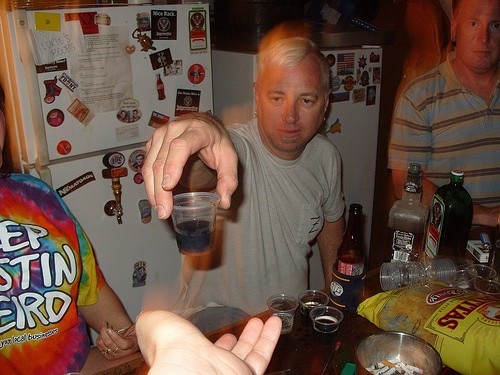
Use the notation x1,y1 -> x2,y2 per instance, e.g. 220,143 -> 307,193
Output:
204,226 -> 500,375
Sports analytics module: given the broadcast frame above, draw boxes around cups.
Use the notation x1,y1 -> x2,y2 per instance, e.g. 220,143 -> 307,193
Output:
380,258 -> 500,298
309,305 -> 344,346
171,192 -> 221,256
267,293 -> 299,334
298,290 -> 329,330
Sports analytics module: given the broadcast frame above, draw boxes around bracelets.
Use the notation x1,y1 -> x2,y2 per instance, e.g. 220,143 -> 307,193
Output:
116,322 -> 133,333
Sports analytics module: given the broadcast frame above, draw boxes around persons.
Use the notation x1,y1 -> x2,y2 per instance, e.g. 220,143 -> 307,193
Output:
133,310 -> 282,375
0,80 -> 137,375
140,37 -> 345,315
388,0 -> 500,232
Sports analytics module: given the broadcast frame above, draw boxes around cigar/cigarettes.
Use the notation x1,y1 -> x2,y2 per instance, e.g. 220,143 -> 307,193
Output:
366,359 -> 424,375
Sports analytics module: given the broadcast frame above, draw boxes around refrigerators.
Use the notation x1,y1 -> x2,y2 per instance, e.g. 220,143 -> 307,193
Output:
213,47 -> 382,290
1,3 -> 212,344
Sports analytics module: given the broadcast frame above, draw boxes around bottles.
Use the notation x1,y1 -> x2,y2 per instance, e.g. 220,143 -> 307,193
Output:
331,204 -> 367,312
425,169 -> 472,257
385,162 -> 429,262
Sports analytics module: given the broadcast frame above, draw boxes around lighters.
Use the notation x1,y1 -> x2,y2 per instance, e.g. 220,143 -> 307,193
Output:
481,233 -> 492,250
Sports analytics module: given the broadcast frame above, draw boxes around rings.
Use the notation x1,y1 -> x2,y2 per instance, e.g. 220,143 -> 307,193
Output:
114,348 -> 118,353
101,350 -> 104,355
106,348 -> 112,354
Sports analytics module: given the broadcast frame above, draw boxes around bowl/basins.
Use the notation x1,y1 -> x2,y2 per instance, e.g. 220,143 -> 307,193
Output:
354,331 -> 442,375
